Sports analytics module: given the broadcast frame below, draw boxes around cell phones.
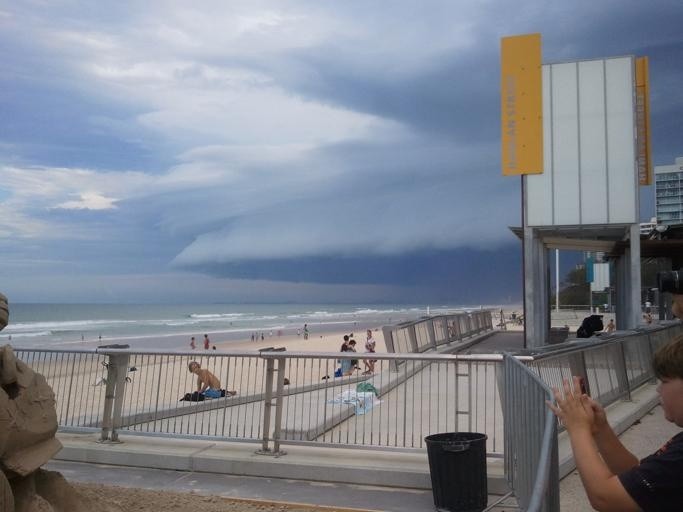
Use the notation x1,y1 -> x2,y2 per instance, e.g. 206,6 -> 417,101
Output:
579,377 -> 587,394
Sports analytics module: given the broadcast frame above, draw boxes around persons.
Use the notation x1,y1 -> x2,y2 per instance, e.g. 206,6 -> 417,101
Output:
643,299 -> 653,323
604,319 -> 615,332
543,333 -> 682,512
511,311 -> 517,319
188,323 -> 377,399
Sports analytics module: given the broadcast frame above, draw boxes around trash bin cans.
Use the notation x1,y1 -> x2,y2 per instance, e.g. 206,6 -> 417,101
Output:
424,432 -> 488,512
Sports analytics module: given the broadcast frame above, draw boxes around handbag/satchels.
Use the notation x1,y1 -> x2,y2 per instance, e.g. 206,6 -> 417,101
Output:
185,392 -> 204,401
